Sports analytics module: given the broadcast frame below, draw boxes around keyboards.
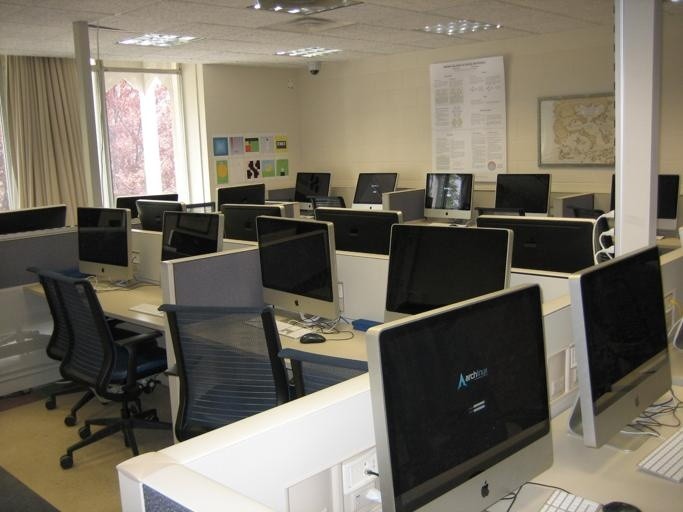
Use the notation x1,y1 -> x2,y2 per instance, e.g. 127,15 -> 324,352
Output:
243,315 -> 312,339
636,427 -> 683,481
127,302 -> 165,318
535,487 -> 606,512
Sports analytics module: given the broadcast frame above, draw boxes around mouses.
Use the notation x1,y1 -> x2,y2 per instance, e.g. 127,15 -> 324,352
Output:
299,333 -> 327,344
602,500 -> 643,511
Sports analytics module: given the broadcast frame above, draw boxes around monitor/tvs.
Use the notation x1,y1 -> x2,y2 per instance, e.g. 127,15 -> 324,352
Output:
160,210 -> 225,264
352,172 -> 400,212
366,282 -> 553,512
134,199 -> 188,231
608,174 -> 680,231
423,172 -> 474,220
568,243 -> 673,453
382,223 -> 514,323
294,171 -> 332,212
219,203 -> 286,242
476,215 -> 601,274
312,206 -> 404,255
216,182 -> 266,212
116,193 -> 179,219
256,214 -> 341,320
76,205 -> 134,281
494,172 -> 551,217
0,204 -> 67,236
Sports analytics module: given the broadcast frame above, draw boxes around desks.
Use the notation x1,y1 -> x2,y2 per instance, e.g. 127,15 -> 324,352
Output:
353,337 -> 679,510
115,347 -> 683,510
25,280 -> 165,335
607,229 -> 681,251
172,308 -> 369,373
403,217 -> 476,227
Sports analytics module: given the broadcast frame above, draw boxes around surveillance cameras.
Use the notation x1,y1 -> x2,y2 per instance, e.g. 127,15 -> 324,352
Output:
308,60 -> 320,74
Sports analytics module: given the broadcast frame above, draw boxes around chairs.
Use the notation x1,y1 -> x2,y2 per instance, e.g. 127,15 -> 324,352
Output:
54,273 -> 174,474
574,206 -> 614,257
309,194 -> 346,220
25,266 -> 140,428
157,303 -> 289,443
279,347 -> 368,395
